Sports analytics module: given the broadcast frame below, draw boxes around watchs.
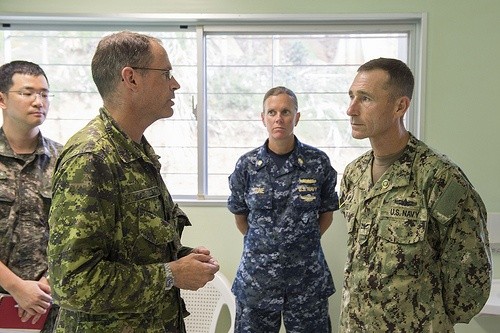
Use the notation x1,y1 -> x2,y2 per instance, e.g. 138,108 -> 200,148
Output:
164,264 -> 174,291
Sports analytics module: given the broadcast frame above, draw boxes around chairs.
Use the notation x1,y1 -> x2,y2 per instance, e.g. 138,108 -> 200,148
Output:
179,271 -> 237,333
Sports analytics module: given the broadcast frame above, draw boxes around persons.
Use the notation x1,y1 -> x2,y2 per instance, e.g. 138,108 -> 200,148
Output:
338,58 -> 491,333
227,86 -> 339,333
47,33 -> 221,333
0,60 -> 64,333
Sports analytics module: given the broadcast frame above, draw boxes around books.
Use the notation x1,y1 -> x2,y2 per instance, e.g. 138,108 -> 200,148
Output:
0,292 -> 51,333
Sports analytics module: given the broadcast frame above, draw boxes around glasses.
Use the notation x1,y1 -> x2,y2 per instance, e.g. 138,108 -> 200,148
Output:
2,89 -> 54,102
122,66 -> 174,81
